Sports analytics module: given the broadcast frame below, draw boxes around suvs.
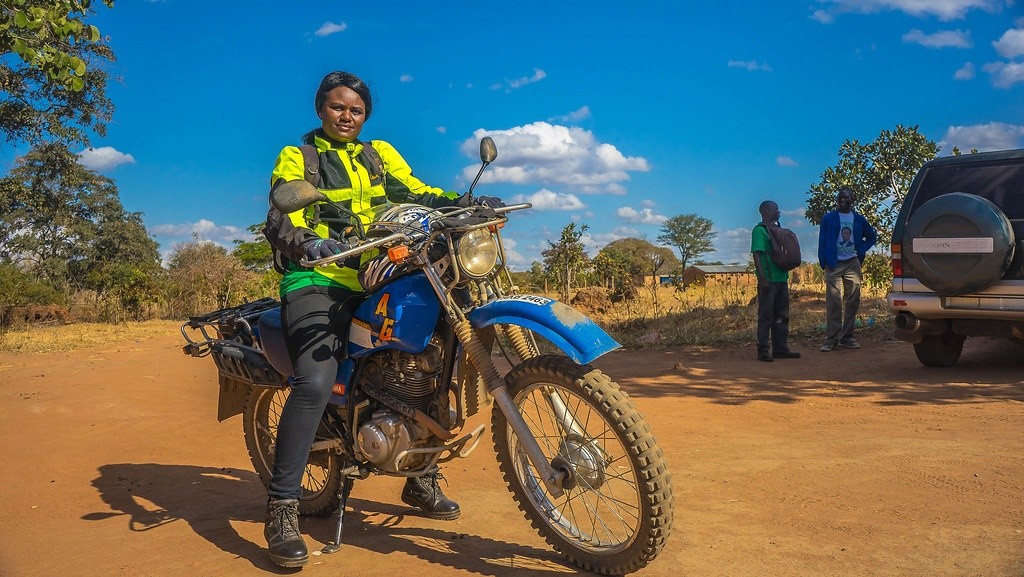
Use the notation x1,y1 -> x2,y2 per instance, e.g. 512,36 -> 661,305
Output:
885,144 -> 1024,370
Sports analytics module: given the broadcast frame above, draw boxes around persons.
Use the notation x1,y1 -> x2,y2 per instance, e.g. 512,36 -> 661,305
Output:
838,226 -> 853,247
818,186 -> 876,351
750,201 -> 801,362
262,71 -> 509,569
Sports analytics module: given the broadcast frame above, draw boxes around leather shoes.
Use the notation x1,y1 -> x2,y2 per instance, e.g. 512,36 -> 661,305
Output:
401,469 -> 461,520
264,497 -> 310,568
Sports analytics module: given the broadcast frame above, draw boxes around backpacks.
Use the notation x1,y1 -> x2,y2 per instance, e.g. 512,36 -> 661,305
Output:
752,220 -> 801,271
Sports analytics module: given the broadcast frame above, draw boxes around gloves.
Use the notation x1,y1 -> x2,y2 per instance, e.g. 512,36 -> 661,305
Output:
303,238 -> 351,268
476,196 -> 510,217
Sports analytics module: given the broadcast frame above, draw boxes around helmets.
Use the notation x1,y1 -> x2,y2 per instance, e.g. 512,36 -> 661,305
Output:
356,202 -> 446,290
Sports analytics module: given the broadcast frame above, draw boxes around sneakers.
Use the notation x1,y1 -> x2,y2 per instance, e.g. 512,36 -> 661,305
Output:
839,339 -> 861,349
820,342 -> 838,352
772,348 -> 801,358
758,354 -> 774,362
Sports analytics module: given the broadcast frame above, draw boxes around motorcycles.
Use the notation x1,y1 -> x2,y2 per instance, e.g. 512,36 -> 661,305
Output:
184,140 -> 676,577
